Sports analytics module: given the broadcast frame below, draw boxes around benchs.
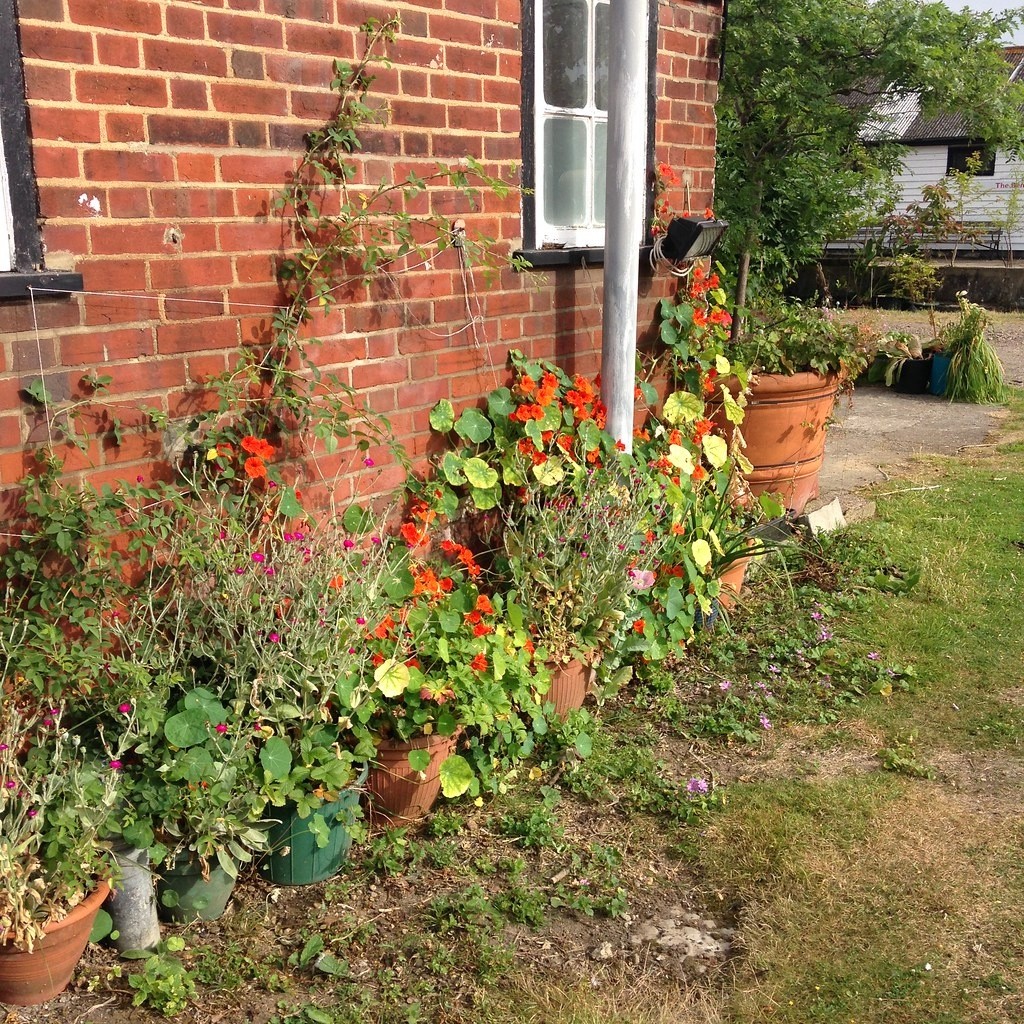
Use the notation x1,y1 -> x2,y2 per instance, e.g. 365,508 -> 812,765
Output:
886,217 -> 1004,261
818,221 -> 885,260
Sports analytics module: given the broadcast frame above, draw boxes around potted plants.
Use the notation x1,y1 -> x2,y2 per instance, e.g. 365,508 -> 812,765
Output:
931,289 -> 1006,406
688,459 -> 824,629
867,329 -> 942,394
701,287 -> 869,525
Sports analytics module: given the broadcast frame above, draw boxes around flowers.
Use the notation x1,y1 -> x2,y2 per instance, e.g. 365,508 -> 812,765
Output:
1,371 -> 711,914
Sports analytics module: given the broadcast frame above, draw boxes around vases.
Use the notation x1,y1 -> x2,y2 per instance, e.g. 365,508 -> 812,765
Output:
0,641 -> 600,1006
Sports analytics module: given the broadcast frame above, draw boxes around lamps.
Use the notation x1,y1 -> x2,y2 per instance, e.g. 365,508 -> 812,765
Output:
643,216 -> 728,262
